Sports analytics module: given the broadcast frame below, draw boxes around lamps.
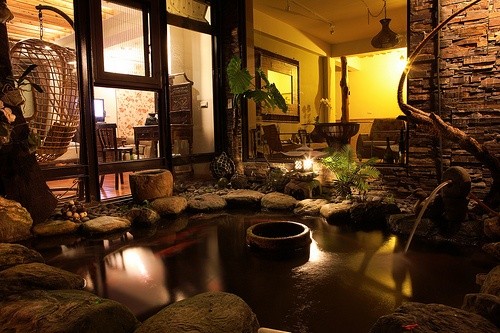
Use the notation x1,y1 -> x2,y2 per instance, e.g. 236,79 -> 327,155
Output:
294,159 -> 313,172
370,0 -> 399,50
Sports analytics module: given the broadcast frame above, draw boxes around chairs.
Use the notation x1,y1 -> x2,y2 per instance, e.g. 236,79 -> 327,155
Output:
262,124 -> 303,154
298,129 -> 309,144
356,118 -> 405,162
96,122 -> 134,191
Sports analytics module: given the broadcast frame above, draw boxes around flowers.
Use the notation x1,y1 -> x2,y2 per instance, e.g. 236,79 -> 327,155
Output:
315,98 -> 334,123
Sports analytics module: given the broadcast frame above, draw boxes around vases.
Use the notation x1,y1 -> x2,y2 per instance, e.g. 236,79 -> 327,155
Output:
309,122 -> 326,143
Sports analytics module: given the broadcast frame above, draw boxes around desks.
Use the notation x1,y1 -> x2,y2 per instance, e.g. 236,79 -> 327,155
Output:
133,124 -> 194,185
315,122 -> 360,156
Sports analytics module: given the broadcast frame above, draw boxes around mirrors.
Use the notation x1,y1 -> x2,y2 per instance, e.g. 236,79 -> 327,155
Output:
254,45 -> 301,124
